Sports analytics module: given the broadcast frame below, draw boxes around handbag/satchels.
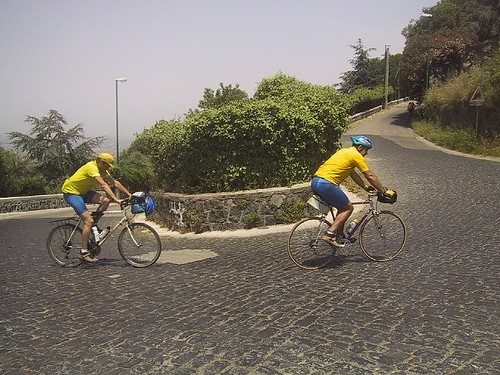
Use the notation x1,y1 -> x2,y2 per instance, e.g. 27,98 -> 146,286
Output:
130,192 -> 146,213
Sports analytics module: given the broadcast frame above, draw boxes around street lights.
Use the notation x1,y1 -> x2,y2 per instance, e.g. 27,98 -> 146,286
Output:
115,78 -> 127,186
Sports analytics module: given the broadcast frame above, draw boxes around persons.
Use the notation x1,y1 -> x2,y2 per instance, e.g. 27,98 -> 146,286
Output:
61,153 -> 131,261
407,102 -> 412,116
311,136 -> 394,247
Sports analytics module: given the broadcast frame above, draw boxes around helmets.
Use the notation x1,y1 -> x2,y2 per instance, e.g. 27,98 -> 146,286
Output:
144,196 -> 154,215
351,135 -> 372,149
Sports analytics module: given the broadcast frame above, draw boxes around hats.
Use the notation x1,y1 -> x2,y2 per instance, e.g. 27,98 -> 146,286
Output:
98,153 -> 115,168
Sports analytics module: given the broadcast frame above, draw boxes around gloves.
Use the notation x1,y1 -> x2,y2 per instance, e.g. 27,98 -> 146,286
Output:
366,186 -> 376,193
383,189 -> 396,198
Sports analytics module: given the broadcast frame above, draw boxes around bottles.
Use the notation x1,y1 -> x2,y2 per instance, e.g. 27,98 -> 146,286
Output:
92,225 -> 100,242
345,218 -> 360,235
98,226 -> 110,239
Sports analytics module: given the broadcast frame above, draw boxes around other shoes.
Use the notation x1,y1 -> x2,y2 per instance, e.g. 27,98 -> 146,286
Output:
340,237 -> 356,244
78,252 -> 98,262
321,232 -> 344,248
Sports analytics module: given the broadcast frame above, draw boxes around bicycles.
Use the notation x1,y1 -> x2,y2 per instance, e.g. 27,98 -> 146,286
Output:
287,182 -> 406,269
46,190 -> 163,271
